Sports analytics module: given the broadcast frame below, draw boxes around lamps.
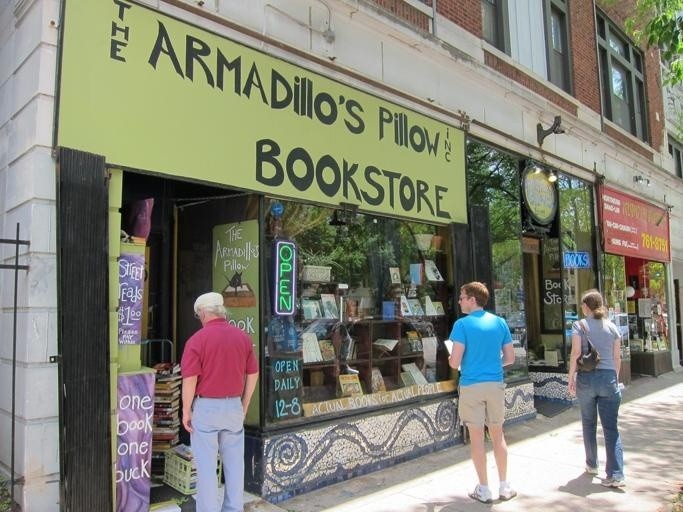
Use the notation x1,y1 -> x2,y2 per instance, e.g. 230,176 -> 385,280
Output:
537,116 -> 565,145
633,176 -> 650,187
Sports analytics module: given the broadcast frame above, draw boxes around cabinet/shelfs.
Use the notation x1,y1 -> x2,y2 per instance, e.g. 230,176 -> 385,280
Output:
267,223 -> 449,403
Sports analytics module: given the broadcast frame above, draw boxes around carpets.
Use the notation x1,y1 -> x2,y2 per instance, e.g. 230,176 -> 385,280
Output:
534,400 -> 570,418
631,375 -> 648,381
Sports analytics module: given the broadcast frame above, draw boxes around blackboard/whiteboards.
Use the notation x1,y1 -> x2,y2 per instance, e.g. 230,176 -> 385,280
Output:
265,351 -> 305,422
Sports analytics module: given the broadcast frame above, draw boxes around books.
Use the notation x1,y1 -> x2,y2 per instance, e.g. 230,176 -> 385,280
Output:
300,260 -> 445,398
151,362 -> 197,489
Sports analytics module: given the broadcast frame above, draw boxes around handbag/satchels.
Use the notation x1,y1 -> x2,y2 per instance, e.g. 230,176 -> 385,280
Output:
574,319 -> 600,372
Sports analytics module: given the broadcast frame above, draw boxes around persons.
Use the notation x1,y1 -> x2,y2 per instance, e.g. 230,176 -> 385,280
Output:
448,280 -> 516,504
180,292 -> 258,512
566,289 -> 627,487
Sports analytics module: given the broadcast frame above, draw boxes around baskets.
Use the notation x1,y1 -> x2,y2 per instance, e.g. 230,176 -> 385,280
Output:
163,444 -> 222,496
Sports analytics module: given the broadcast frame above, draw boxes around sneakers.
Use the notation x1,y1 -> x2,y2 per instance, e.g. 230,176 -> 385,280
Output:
600,477 -> 625,487
467,484 -> 491,504
498,485 -> 516,501
585,463 -> 599,475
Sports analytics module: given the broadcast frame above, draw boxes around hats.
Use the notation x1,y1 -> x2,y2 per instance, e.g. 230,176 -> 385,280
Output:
193,291 -> 223,312
